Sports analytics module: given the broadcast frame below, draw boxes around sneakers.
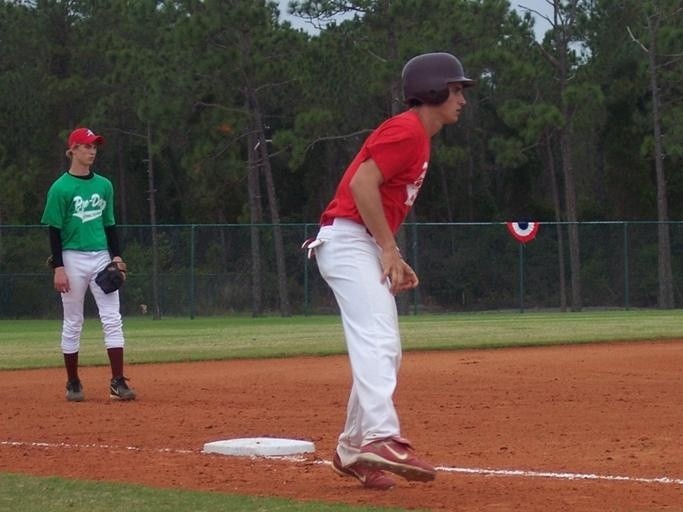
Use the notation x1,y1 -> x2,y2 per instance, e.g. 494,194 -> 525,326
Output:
358,437 -> 437,481
66,379 -> 84,401
332,452 -> 395,490
110,377 -> 136,399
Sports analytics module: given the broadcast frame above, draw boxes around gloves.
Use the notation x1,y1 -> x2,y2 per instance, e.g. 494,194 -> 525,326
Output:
302,238 -> 321,259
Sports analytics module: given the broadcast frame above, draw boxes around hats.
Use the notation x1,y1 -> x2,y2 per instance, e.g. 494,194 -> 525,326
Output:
68,128 -> 103,145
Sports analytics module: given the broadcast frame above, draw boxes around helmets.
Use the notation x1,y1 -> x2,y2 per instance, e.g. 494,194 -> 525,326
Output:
402,53 -> 478,105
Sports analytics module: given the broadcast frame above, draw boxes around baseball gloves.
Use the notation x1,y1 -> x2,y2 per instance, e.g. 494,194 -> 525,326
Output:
95,263 -> 126,294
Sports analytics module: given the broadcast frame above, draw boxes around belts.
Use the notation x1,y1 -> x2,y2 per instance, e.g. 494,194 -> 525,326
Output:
321,216 -> 373,237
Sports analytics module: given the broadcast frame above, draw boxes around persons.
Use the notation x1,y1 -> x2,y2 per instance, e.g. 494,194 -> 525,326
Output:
301,52 -> 477,491
41,129 -> 136,400
